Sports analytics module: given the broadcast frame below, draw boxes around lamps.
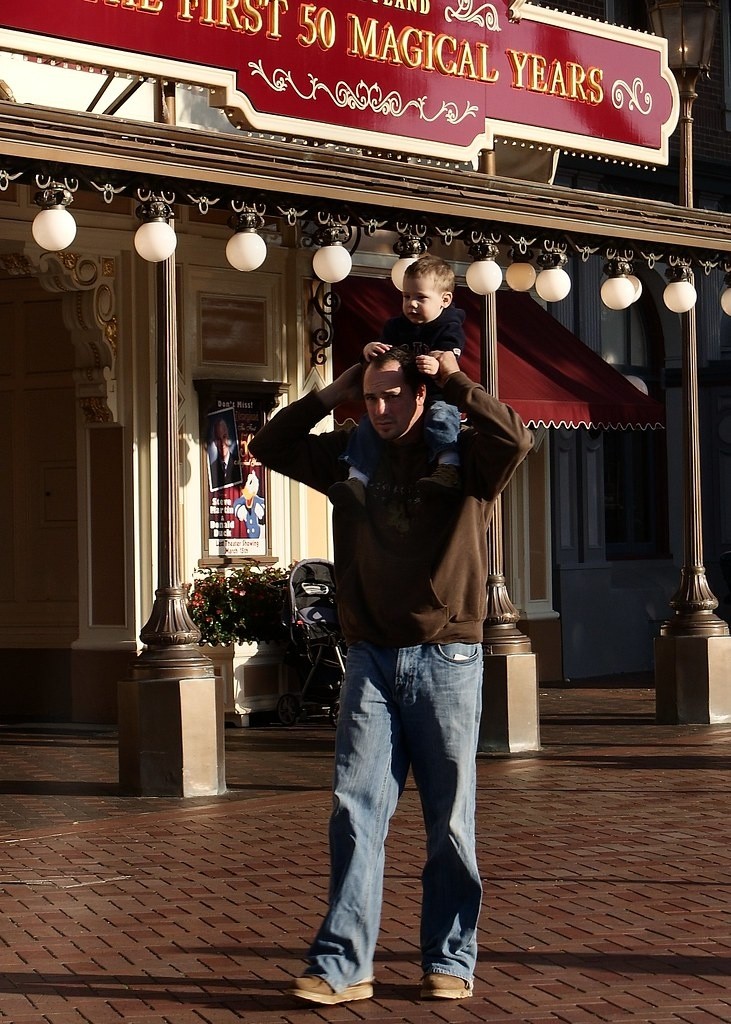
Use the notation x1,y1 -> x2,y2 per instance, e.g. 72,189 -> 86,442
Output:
624,258 -> 643,303
391,230 -> 427,292
465,238 -> 503,295
312,220 -> 353,283
32,181 -> 77,250
506,243 -> 537,291
535,247 -> 572,302
663,260 -> 697,313
720,271 -> 731,316
225,206 -> 268,271
134,194 -> 178,262
600,251 -> 635,310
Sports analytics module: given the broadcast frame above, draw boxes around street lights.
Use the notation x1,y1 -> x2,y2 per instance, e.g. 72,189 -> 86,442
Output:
646,0 -> 730,635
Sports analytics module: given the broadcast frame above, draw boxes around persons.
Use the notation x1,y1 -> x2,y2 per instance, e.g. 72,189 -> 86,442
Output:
327,256 -> 465,517
248,350 -> 536,1004
211,419 -> 241,487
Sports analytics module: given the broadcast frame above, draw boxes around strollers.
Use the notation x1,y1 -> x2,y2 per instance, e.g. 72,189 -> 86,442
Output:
266,558 -> 347,726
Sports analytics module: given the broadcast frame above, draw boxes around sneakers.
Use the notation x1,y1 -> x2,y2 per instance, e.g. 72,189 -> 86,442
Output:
419,972 -> 471,999
327,478 -> 366,519
288,974 -> 374,1004
417,462 -> 462,496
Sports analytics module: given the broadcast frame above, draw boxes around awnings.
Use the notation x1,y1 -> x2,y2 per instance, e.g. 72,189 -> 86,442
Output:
334,279 -> 666,429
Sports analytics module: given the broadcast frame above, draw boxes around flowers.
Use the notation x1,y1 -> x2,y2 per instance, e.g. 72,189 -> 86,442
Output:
189,560 -> 297,647
